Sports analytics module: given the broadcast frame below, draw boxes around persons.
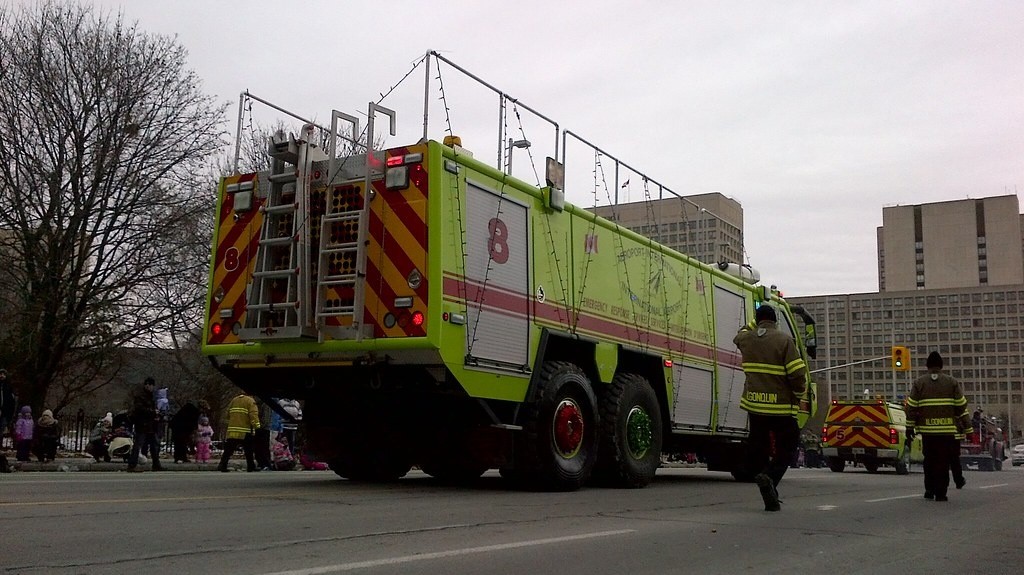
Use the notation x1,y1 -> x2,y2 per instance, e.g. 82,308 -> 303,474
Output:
154,388 -> 169,441
15,406 -> 35,463
33,409 -> 61,463
664,451 -> 707,463
218,388 -> 260,471
904,351 -> 966,500
791,429 -> 828,469
194,416 -> 214,463
0,369 -> 12,472
733,305 -> 809,512
948,409 -> 975,490
88,412 -> 113,462
253,395 -> 305,470
171,400 -> 210,463
128,377 -> 168,472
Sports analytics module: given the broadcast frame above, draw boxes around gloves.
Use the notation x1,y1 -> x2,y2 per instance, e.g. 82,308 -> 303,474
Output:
15,434 -> 23,441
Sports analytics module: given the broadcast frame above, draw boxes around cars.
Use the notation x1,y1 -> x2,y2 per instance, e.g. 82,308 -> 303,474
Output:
1011,445 -> 1024,466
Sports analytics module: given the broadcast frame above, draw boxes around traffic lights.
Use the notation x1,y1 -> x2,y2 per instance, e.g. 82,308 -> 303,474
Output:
892,347 -> 905,370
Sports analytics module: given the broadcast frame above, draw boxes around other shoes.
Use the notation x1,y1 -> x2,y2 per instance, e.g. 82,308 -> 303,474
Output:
956,476 -> 966,489
755,473 -> 782,511
924,489 -> 948,501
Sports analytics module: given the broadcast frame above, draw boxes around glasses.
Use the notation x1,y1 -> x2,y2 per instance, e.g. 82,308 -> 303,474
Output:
144,382 -> 152,386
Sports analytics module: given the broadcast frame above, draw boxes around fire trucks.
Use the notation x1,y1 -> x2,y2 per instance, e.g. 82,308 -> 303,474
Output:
199,52 -> 820,492
959,407 -> 1008,471
820,399 -> 925,475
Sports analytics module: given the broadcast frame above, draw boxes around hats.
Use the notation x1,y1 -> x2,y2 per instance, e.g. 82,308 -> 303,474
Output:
42,409 -> 53,419
21,406 -> 32,413
926,350 -> 943,370
158,387 -> 168,397
756,305 -> 777,324
200,416 -> 209,424
103,412 -> 113,426
